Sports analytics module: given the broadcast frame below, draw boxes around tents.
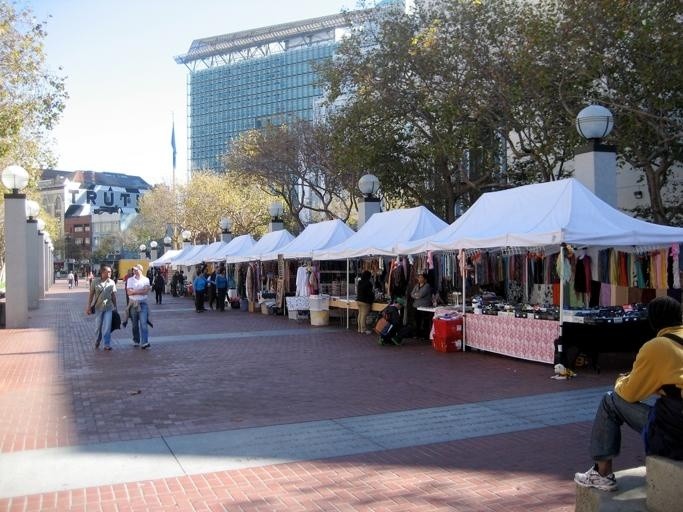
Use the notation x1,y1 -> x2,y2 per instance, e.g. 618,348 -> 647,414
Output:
311,206 -> 451,329
148,206 -> 356,284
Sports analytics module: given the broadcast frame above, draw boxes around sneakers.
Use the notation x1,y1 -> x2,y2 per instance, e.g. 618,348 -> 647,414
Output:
377,335 -> 402,346
574,463 -> 618,492
95,341 -> 112,350
133,342 -> 150,350
358,330 -> 372,335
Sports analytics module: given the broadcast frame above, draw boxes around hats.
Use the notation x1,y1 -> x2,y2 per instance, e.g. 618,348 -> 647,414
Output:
648,297 -> 683,329
396,298 -> 405,305
133,264 -> 144,271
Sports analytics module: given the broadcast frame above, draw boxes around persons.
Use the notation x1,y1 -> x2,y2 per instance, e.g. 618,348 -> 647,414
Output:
356,270 -> 375,335
68,268 -> 119,290
126,264 -> 153,349
411,274 -> 431,344
147,267 -> 186,305
192,264 -> 229,313
573,293 -> 683,493
86,265 -> 118,350
122,268 -> 134,305
375,298 -> 411,346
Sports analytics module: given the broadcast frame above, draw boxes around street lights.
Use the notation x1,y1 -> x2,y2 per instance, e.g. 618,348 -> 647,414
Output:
1,165 -> 54,327
181,230 -> 192,248
358,174 -> 381,231
163,236 -> 172,253
219,216 -> 231,241
575,104 -> 616,213
269,203 -> 283,233
150,241 -> 157,261
139,243 -> 146,258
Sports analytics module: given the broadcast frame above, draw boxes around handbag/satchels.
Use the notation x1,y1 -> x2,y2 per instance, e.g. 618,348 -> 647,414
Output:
643,394 -> 682,462
89,305 -> 96,315
373,317 -> 394,336
